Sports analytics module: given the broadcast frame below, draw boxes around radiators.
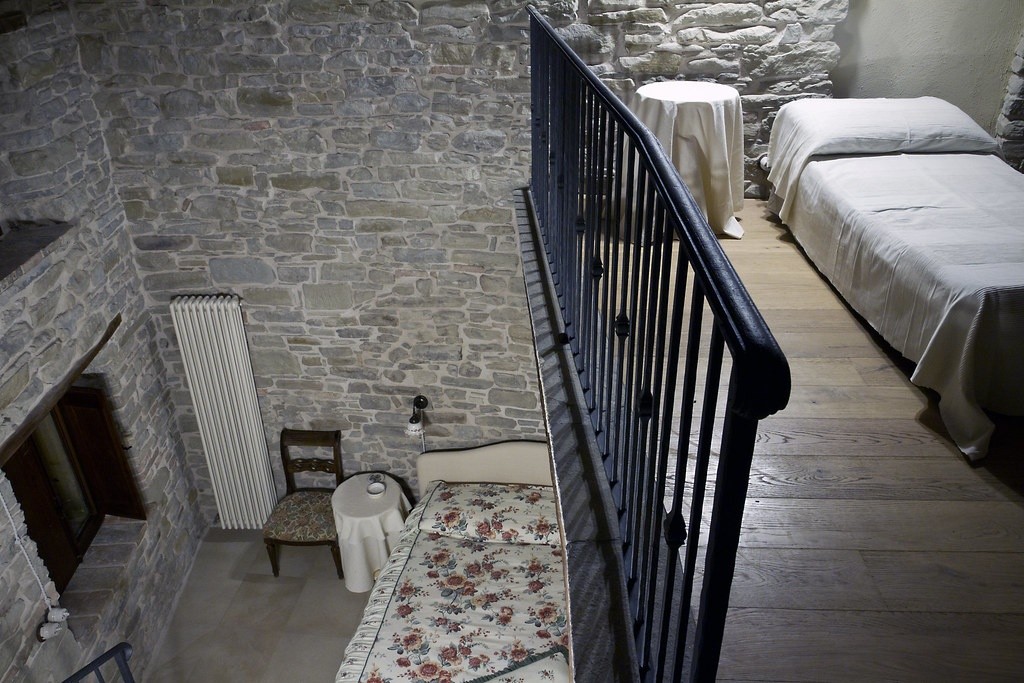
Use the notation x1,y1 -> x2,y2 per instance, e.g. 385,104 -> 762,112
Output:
170,296 -> 278,528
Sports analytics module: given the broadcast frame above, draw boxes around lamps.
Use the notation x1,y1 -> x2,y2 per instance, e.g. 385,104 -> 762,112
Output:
403,395 -> 429,452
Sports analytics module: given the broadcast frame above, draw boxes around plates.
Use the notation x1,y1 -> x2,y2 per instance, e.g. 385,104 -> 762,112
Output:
367,473 -> 384,483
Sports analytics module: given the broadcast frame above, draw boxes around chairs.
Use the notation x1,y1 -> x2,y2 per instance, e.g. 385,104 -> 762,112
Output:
262,427 -> 344,581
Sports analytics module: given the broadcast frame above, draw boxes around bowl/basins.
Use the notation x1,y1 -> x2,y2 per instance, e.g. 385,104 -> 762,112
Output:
366,483 -> 385,499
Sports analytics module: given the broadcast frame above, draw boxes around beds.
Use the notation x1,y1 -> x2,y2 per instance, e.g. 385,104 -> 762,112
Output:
770,152 -> 1024,462
336,440 -> 573,683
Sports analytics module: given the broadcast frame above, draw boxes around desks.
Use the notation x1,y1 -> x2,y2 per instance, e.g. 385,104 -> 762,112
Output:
601,81 -> 745,247
331,472 -> 412,593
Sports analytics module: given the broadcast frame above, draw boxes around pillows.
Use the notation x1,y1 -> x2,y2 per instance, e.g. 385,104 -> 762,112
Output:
767,96 -> 999,225
406,479 -> 561,545
